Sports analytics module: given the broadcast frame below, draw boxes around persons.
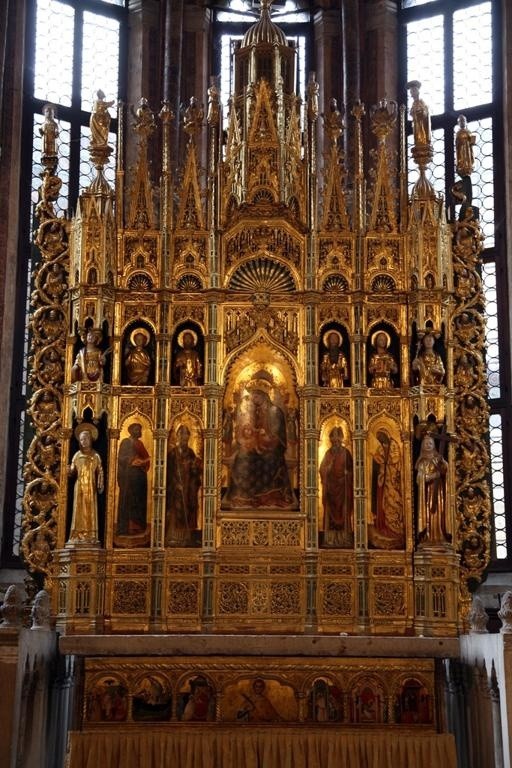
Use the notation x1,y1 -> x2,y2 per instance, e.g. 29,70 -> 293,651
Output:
319,427 -> 449,549
230,380 -> 295,509
236,680 -> 280,725
71,333 -> 200,387
70,424 -> 203,544
321,332 -> 445,389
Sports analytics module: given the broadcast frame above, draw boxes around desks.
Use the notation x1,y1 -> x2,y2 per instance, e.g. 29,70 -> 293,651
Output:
54,632 -> 462,767
